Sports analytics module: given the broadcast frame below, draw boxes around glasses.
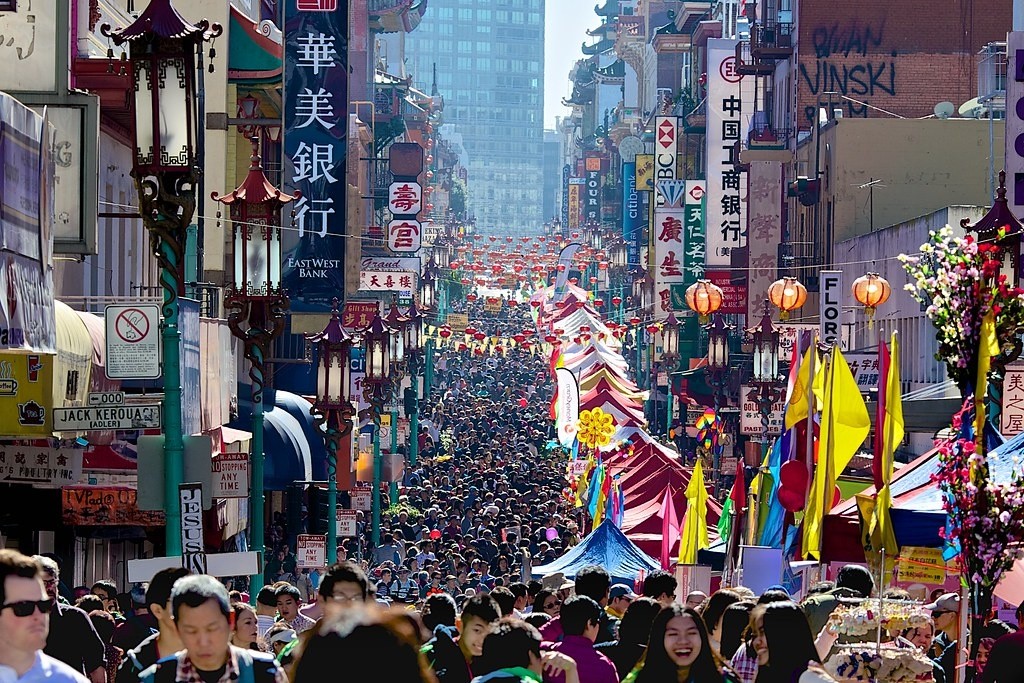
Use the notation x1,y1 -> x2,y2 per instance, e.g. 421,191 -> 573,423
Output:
277,640 -> 288,645
434,577 -> 441,580
670,595 -> 676,600
323,593 -> 362,604
615,596 -> 632,604
931,609 -> 958,618
398,571 -> 407,574
43,579 -> 55,588
0,600 -> 50,616
90,592 -> 109,600
543,601 -> 561,609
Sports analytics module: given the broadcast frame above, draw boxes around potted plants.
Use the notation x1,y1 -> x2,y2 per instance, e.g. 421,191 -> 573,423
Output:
672,86 -> 706,127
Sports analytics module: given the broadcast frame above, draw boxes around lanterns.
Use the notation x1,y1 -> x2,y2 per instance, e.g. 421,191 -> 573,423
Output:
440,315 -> 659,354
852,272 -> 891,317
768,277 -> 807,320
684,279 -> 723,323
424,123 -> 434,210
440,232 -> 660,309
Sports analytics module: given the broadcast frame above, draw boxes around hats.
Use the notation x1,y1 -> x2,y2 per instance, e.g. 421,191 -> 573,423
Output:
270,629 -> 297,645
540,571 -> 575,592
445,575 -> 456,581
924,593 -> 960,612
256,585 -> 277,607
131,583 -> 150,604
396,565 -> 408,573
337,546 -> 348,553
610,584 -> 639,600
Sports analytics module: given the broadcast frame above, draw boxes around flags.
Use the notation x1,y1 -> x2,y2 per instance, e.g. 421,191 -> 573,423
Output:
656,485 -> 680,572
808,347 -> 871,561
678,457 -> 709,564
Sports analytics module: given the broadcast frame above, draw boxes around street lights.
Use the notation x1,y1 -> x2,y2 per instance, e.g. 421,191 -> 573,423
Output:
741,298 -> 785,466
300,294 -> 364,566
98,1 -> 224,556
355,299 -> 403,553
634,271 -> 655,390
702,310 -> 734,501
658,311 -> 687,443
210,134 -> 303,606
385,292 -> 439,506
607,259 -> 626,325
960,170 -> 1024,451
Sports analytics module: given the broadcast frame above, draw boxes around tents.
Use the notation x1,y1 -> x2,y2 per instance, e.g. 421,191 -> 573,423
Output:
823,421 -> 1008,561
530,280 -> 728,588
890,421 -> 1024,549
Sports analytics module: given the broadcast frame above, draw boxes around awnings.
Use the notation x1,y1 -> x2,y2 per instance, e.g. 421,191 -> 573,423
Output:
275,389 -> 330,481
261,407 -> 312,490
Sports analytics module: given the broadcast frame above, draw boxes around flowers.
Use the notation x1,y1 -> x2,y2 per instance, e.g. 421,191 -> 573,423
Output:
897,223 -> 1024,401
930,437 -> 1024,683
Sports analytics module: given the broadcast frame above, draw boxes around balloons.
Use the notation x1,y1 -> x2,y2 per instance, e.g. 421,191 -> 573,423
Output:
778,484 -> 805,512
430,529 -> 440,539
780,459 -> 808,493
830,485 -> 841,509
520,398 -> 526,407
546,527 -> 557,542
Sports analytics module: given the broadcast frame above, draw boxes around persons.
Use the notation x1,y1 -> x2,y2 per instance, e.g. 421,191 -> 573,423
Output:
0,338 -> 1024,683
450,296 -> 540,345
